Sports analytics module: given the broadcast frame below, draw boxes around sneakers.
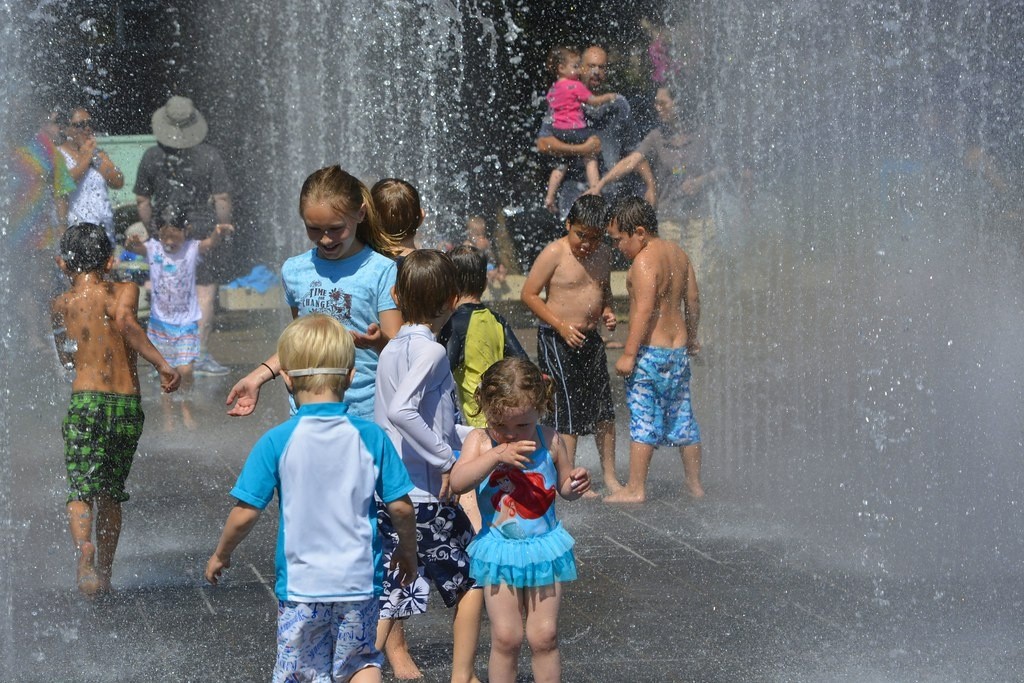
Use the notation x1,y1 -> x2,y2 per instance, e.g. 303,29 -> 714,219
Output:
193,354 -> 233,378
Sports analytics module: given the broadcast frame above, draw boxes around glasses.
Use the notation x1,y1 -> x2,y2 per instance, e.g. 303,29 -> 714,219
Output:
69,119 -> 98,129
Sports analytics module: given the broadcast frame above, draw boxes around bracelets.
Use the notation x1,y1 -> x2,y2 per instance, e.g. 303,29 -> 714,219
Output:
262,363 -> 276,379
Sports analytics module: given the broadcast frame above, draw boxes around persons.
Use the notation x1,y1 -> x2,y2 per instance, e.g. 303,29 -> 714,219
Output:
206,314 -> 418,683
16,94 -> 236,432
48,222 -> 180,606
370,177 -> 592,683
226,166 -> 406,425
537,15 -> 727,275
603,196 -> 709,502
521,194 -> 625,496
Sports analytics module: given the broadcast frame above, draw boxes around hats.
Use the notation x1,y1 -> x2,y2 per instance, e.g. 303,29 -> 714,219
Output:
151,95 -> 209,150
